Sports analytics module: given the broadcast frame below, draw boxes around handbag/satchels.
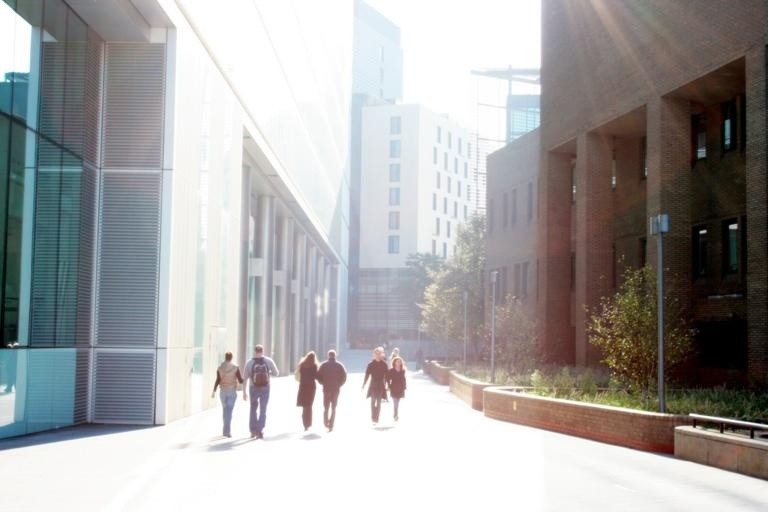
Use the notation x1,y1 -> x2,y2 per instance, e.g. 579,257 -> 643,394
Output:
295,368 -> 300,382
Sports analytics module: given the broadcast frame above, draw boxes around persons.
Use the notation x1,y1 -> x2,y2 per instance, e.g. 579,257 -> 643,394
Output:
208,350 -> 246,437
360,344 -> 390,423
314,349 -> 348,434
388,348 -> 401,363
243,344 -> 280,438
384,356 -> 409,422
293,349 -> 318,432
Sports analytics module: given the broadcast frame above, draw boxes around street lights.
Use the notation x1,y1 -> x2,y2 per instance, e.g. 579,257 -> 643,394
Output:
648,210 -> 672,413
489,271 -> 499,384
461,291 -> 470,377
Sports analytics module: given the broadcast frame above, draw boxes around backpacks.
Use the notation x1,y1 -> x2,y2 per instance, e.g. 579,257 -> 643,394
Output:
252,357 -> 269,385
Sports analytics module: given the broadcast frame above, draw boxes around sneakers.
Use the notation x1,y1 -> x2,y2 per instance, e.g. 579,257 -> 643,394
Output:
223,433 -> 231,437
251,430 -> 263,438
